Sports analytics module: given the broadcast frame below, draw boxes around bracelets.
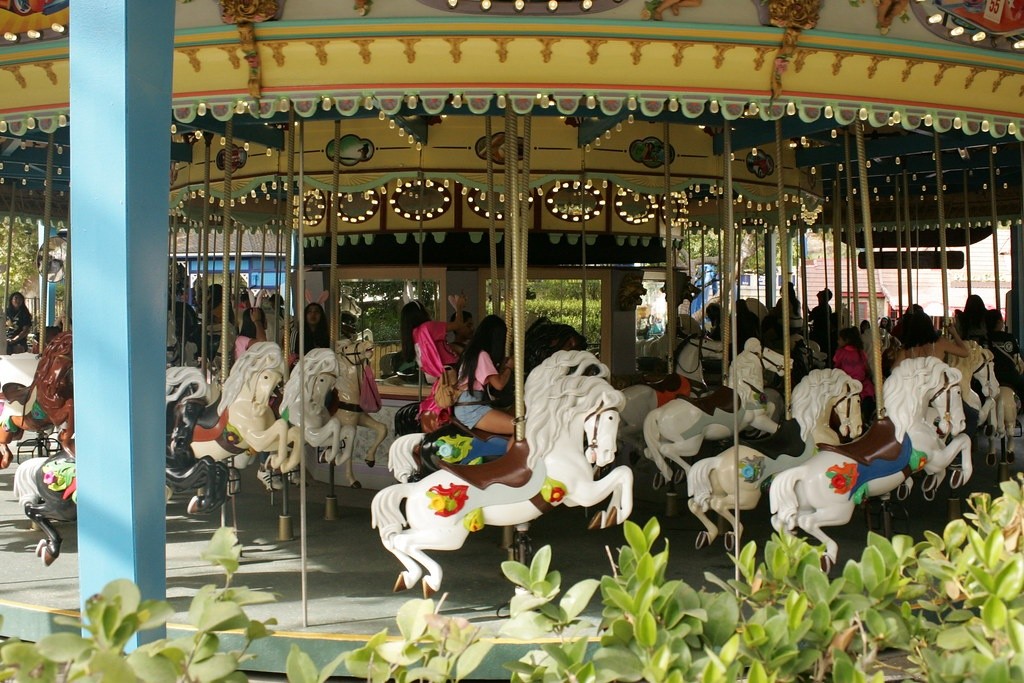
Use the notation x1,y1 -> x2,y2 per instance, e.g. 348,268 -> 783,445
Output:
253,319 -> 262,323
504,366 -> 512,371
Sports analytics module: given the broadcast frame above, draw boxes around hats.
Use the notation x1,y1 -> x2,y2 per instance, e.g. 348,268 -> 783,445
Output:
268,294 -> 283,302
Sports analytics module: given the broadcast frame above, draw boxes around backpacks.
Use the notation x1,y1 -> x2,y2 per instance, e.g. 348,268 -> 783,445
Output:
434,350 -> 486,408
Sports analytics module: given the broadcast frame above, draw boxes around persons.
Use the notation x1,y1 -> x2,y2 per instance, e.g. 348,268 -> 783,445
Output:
833,295 -> 1024,471
453,315 -> 516,452
400,294 -> 474,387
5,292 -> 32,355
167,280 -> 332,489
706,282 -> 832,365
636,305 -> 660,340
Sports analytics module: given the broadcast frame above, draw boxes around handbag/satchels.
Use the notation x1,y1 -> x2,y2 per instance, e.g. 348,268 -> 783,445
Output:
359,359 -> 382,413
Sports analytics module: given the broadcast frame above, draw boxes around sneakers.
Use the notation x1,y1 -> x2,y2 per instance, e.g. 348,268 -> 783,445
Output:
256,468 -> 283,490
287,470 -> 300,484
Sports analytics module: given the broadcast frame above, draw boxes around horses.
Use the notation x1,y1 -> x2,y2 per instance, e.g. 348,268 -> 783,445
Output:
0,298 -> 1024,601
